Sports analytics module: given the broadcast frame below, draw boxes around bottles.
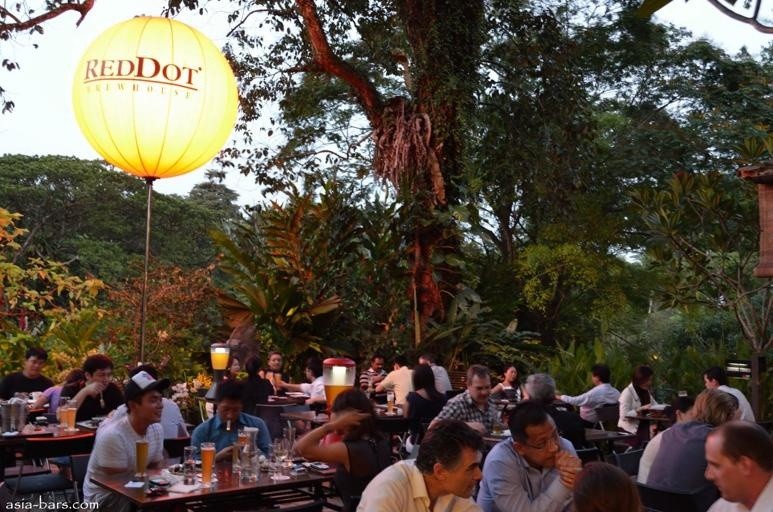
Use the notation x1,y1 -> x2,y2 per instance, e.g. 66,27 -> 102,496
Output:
240,428 -> 259,484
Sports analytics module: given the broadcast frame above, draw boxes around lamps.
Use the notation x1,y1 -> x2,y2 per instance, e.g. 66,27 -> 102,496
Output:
69,18 -> 235,370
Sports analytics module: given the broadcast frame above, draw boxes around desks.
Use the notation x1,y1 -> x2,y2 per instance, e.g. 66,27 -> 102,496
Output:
1,367 -> 771,510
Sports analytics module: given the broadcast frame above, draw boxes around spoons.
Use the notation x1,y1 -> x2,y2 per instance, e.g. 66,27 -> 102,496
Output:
99,391 -> 105,408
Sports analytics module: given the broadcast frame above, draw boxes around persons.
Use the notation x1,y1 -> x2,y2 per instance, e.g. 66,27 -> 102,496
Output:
0,346 -> 772,512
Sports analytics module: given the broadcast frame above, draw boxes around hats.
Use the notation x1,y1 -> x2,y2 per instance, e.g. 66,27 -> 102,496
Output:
123,371 -> 171,403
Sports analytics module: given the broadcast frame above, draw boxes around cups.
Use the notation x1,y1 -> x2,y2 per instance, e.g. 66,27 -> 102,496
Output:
136,435 -> 148,474
28,392 -> 41,400
200,442 -> 213,481
236,429 -> 245,443
232,441 -> 241,472
386,391 -> 394,416
492,422 -> 502,434
367,376 -> 373,389
678,390 -> 688,399
183,446 -> 196,478
267,427 -> 296,481
59,397 -> 69,423
66,399 -> 77,429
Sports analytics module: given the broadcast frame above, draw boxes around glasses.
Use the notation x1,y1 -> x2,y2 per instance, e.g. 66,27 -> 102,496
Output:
521,430 -> 560,451
